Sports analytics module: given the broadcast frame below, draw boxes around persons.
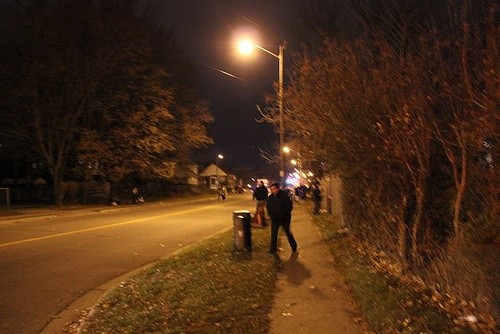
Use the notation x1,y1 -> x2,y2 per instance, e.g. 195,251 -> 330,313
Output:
312,186 -> 321,214
218,186 -> 227,199
267,183 -> 297,254
295,185 -> 307,198
254,181 -> 268,226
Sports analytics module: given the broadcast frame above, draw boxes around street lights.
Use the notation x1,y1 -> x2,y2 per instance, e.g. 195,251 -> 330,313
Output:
283,147 -> 303,175
238,40 -> 284,166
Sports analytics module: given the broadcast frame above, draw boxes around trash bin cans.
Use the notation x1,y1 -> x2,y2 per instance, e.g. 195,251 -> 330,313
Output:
233,210 -> 252,252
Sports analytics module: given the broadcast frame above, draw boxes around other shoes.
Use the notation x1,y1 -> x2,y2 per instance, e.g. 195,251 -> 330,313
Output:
293,247 -> 296,252
269,248 -> 276,253
